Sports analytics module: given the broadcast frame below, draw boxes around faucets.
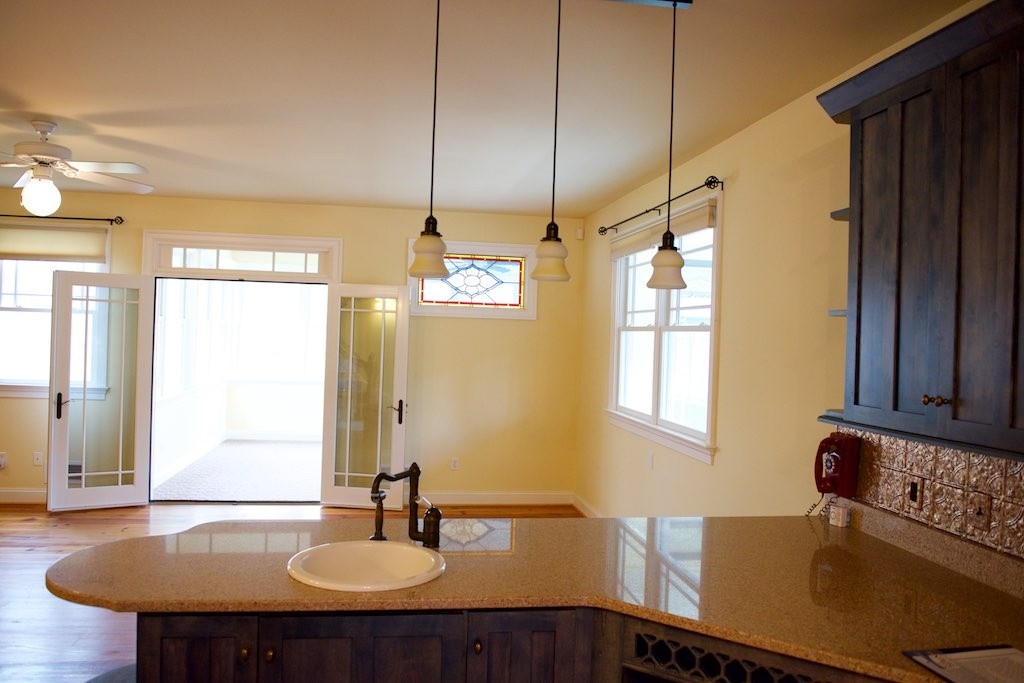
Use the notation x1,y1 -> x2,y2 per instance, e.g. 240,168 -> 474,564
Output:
367,461 -> 441,549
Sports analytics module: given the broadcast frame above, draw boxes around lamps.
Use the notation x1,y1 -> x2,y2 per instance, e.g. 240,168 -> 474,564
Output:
22,165 -> 62,216
404,0 -> 452,278
530,0 -> 570,281
646,0 -> 687,288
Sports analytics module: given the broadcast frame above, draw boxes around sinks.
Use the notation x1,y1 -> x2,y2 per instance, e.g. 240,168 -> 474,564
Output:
287,540 -> 446,594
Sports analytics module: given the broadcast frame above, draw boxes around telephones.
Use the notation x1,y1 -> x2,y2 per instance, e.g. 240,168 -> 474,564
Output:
814,432 -> 857,497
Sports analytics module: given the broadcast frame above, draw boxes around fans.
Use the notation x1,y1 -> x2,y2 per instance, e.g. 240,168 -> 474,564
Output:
0,121 -> 154,194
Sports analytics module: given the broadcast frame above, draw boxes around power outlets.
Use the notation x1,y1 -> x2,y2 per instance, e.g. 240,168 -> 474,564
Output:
33,452 -> 42,466
450,456 -> 460,470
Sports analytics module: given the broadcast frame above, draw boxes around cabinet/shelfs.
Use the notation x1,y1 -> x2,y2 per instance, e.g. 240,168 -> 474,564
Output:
816,1 -> 1023,462
468,606 -> 597,683
135,612 -> 467,683
